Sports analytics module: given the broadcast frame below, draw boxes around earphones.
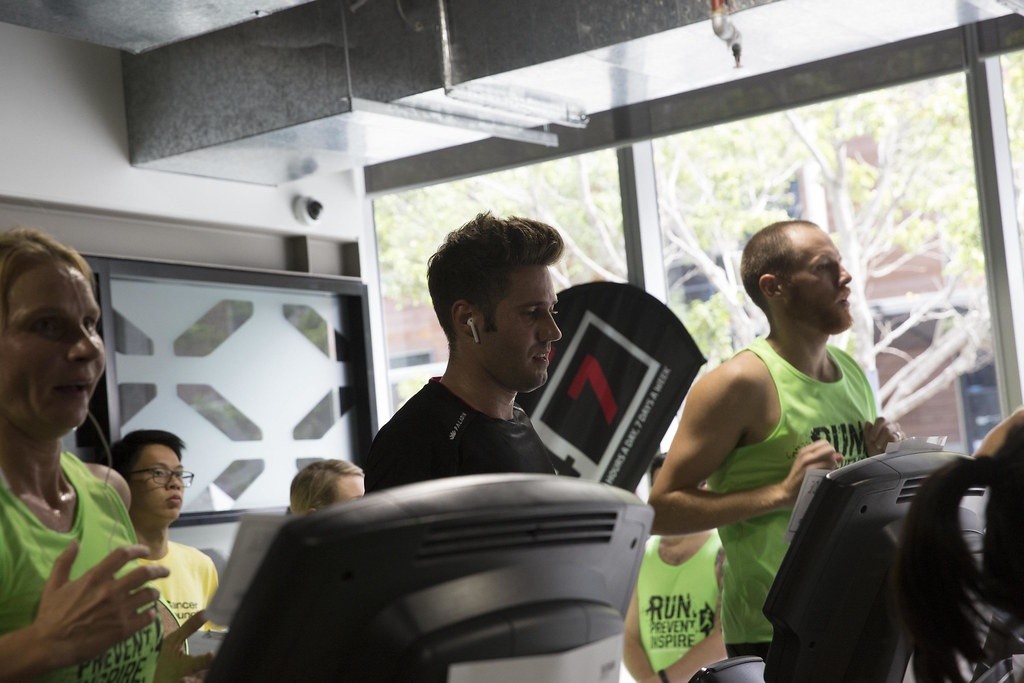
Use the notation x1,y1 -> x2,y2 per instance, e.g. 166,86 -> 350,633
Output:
467,317 -> 480,343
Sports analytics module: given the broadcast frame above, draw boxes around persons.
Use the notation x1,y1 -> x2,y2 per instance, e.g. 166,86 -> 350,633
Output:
361,209 -> 563,491
646,219 -> 905,663
1,228 -> 214,683
286,458 -> 366,516
107,430 -> 228,632
622,453 -> 730,682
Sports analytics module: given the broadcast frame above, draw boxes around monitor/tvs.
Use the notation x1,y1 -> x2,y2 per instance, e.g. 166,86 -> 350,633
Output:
762,446 -> 988,683
202,475 -> 655,683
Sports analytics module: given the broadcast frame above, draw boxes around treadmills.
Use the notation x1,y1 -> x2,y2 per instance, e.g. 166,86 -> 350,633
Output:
692,446 -> 991,683
202,473 -> 653,683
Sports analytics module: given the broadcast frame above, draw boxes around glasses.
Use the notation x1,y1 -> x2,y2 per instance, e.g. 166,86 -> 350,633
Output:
130,468 -> 194,486
